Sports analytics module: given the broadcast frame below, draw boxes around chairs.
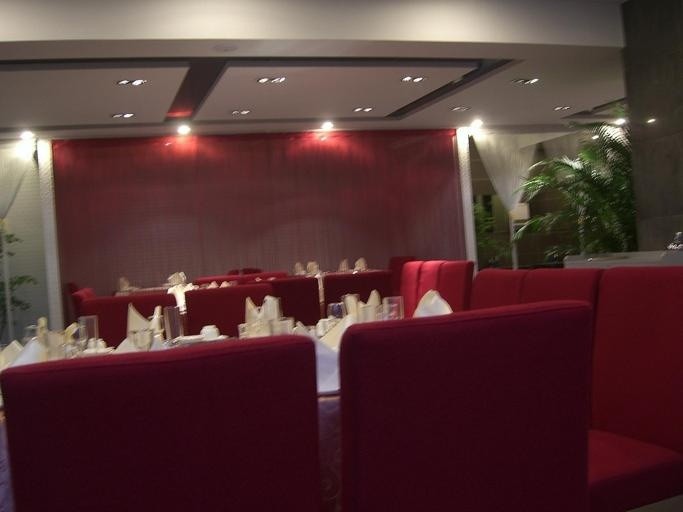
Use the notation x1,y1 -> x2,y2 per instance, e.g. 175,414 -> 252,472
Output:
0,255 -> 683,512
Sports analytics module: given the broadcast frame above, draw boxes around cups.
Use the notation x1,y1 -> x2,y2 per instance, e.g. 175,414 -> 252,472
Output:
131,306 -> 183,353
327,293 -> 403,324
200,324 -> 220,340
22,315 -> 99,361
237,295 -> 294,340
